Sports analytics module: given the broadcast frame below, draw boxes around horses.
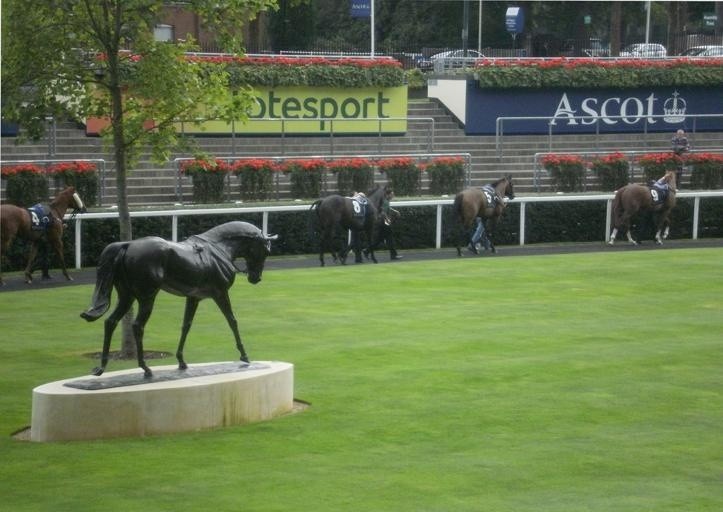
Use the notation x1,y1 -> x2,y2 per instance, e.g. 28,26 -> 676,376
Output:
451,171 -> 516,257
307,182 -> 388,267
78,219 -> 279,375
0,184 -> 89,289
608,167 -> 679,247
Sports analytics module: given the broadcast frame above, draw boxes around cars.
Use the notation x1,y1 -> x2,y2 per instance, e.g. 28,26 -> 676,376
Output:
679,46 -> 723,56
418,50 -> 485,69
567,39 -> 606,56
619,44 -> 666,57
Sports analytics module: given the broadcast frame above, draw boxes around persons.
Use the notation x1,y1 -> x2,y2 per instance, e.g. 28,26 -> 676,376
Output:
671,129 -> 689,176
347,190 -> 404,260
467,217 -> 490,255
25,239 -> 53,284
